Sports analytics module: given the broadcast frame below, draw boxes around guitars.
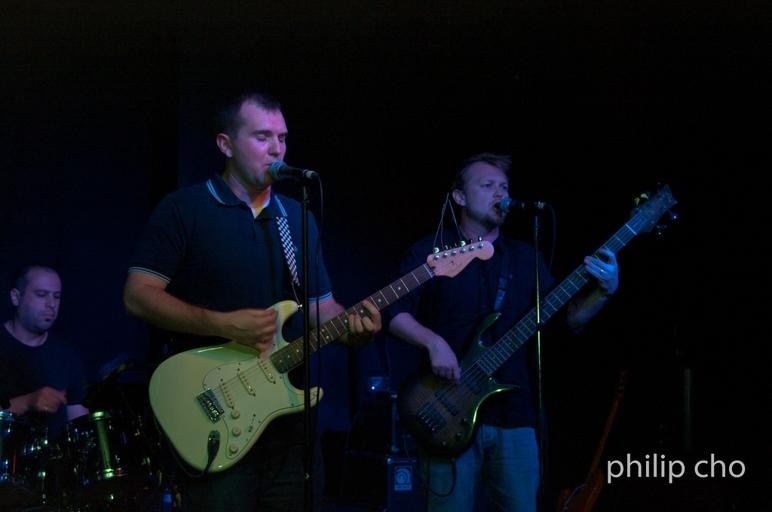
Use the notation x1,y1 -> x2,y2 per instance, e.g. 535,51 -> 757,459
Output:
403,187 -> 678,456
148,238 -> 494,471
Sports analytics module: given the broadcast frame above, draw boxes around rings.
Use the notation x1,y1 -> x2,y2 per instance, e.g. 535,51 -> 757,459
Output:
598,266 -> 606,274
42,406 -> 49,412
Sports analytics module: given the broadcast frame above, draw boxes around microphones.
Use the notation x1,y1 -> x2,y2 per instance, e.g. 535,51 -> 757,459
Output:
497,197 -> 548,215
267,159 -> 317,183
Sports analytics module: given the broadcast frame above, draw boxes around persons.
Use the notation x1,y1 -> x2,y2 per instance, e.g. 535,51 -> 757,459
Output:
0,265 -> 75,418
122,88 -> 384,510
387,150 -> 620,511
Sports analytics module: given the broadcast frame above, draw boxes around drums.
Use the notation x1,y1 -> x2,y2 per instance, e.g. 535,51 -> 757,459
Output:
61,412 -> 149,490
1,411 -> 18,482
